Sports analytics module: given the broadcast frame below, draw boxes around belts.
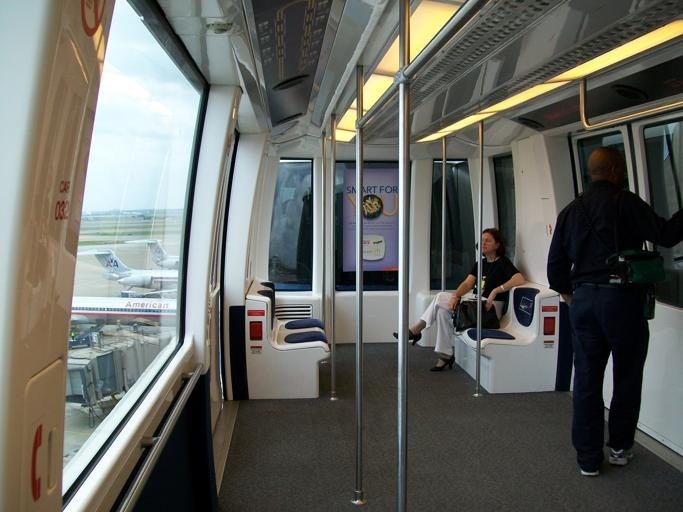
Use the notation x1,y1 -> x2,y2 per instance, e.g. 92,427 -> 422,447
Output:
583,283 -> 624,289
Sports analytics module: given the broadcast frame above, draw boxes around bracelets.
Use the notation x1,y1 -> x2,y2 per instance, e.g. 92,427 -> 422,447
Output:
501,285 -> 505,292
452,295 -> 459,300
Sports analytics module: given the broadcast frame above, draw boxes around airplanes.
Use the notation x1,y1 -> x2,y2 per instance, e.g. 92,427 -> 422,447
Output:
71,239 -> 179,318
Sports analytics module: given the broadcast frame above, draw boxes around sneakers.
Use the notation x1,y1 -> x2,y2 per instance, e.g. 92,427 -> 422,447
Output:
607,448 -> 633,467
580,469 -> 599,476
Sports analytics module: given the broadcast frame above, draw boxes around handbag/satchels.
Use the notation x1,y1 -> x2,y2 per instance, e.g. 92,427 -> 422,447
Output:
451,300 -> 499,332
623,250 -> 664,284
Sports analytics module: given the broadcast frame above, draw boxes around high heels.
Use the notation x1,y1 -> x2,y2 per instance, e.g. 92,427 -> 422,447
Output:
392,330 -> 420,346
429,357 -> 453,371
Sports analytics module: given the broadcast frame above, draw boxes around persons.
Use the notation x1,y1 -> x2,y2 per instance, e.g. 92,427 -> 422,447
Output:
546,148 -> 681,478
393,228 -> 527,372
71,331 -> 75,340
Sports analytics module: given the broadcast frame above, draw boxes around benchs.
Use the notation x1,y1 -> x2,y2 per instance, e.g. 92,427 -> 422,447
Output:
451,279 -> 562,394
242,279 -> 329,401
